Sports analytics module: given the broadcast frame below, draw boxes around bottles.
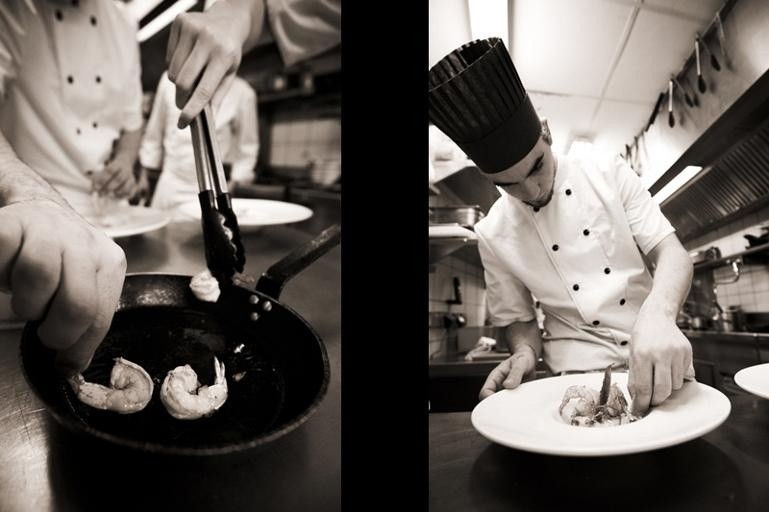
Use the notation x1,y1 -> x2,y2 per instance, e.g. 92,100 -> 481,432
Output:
426,38 -> 540,174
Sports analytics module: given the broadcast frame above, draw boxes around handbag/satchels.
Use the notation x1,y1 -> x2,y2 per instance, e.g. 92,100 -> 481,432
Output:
514,342 -> 538,376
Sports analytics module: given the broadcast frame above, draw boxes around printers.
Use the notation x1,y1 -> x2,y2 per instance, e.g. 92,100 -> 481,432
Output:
666,14 -> 730,127
187,100 -> 250,284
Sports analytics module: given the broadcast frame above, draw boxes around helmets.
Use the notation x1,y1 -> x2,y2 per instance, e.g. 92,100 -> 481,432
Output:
470,370 -> 733,457
77,202 -> 169,238
733,363 -> 769,401
180,197 -> 314,239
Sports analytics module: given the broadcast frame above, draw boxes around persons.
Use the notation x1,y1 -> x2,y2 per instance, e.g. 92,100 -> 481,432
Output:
0,0 -> 144,372
137,69 -> 259,207
474,118 -> 694,416
166,0 -> 341,129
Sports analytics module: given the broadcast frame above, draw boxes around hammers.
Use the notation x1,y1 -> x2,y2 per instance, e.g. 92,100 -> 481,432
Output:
560,382 -> 629,425
160,356 -> 231,420
63,355 -> 157,415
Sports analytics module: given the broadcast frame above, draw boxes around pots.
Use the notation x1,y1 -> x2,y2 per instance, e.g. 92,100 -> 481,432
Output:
18,223 -> 343,457
711,299 -> 746,333
